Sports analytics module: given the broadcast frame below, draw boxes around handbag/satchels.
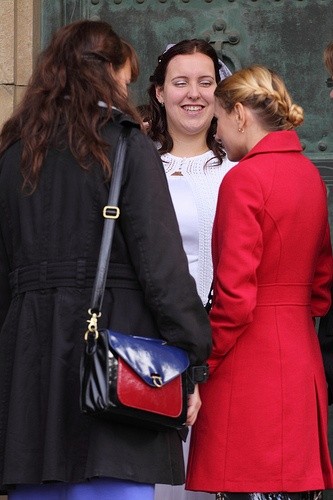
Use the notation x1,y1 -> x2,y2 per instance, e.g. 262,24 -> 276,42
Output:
79,329 -> 192,434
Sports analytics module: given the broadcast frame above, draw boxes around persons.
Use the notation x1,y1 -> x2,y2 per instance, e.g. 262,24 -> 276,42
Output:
185,64 -> 333,500
0,19 -> 210,500
308,44 -> 333,406
130,37 -> 240,500
